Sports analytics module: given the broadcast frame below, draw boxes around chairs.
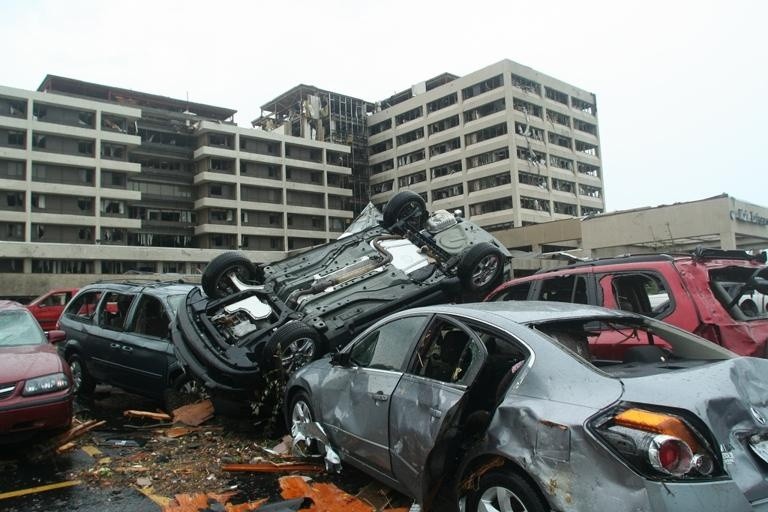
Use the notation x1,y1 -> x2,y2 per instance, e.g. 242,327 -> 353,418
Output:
439,329 -> 470,360
742,300 -> 760,317
110,301 -> 166,335
